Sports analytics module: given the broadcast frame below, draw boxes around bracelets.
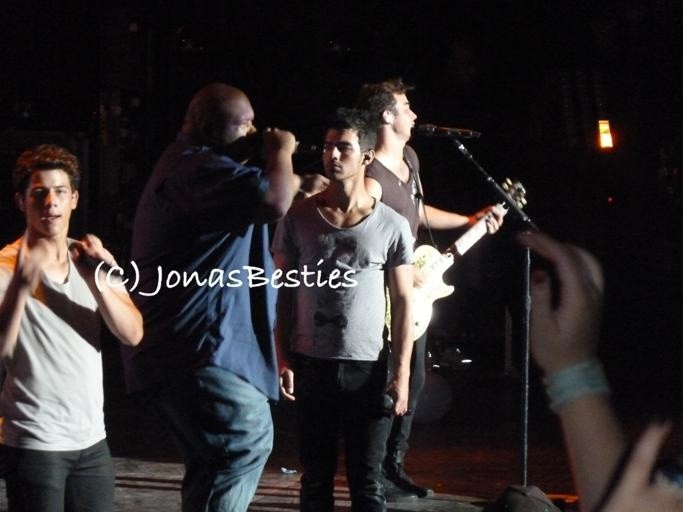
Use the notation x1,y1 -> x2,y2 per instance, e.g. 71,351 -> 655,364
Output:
538,356 -> 616,413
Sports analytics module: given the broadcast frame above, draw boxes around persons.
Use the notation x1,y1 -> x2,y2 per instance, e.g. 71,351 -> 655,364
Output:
128,83 -> 302,512
0,140 -> 147,512
514,230 -> 683,512
354,76 -> 504,503
273,106 -> 414,512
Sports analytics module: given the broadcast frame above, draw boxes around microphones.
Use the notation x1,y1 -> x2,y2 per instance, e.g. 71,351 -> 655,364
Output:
260,131 -> 323,155
68,242 -> 121,278
418,124 -> 482,138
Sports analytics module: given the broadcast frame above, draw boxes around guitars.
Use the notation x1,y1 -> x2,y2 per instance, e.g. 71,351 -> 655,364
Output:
387,177 -> 528,344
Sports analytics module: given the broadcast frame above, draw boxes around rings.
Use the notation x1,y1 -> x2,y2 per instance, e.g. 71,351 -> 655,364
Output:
655,467 -> 683,497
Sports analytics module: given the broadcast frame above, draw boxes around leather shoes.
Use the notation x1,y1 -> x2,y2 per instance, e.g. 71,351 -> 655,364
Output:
377,465 -> 436,505
